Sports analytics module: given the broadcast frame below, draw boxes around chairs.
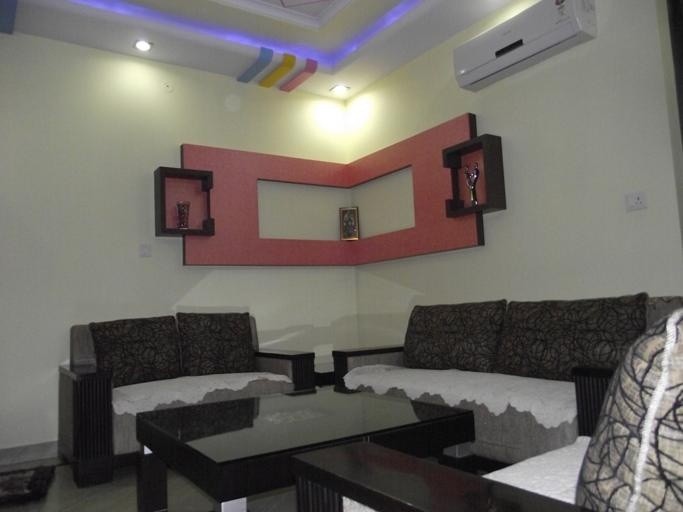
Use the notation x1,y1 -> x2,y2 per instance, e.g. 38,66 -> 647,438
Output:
292,366 -> 683,512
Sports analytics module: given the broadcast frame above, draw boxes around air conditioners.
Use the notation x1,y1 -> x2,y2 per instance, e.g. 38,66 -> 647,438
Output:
453,0 -> 598,92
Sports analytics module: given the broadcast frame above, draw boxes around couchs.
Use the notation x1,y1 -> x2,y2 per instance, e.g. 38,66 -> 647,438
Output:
332,296 -> 683,465
59,316 -> 315,488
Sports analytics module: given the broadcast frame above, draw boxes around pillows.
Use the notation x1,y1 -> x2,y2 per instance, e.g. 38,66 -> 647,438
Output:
491,292 -> 648,382
176,312 -> 259,375
576,307 -> 683,512
88,316 -> 181,387
403,299 -> 507,373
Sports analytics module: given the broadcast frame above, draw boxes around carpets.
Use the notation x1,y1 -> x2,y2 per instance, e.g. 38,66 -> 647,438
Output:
0,465 -> 55,507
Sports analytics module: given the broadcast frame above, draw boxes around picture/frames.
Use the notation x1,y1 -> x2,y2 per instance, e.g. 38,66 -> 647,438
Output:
340,207 -> 359,241
